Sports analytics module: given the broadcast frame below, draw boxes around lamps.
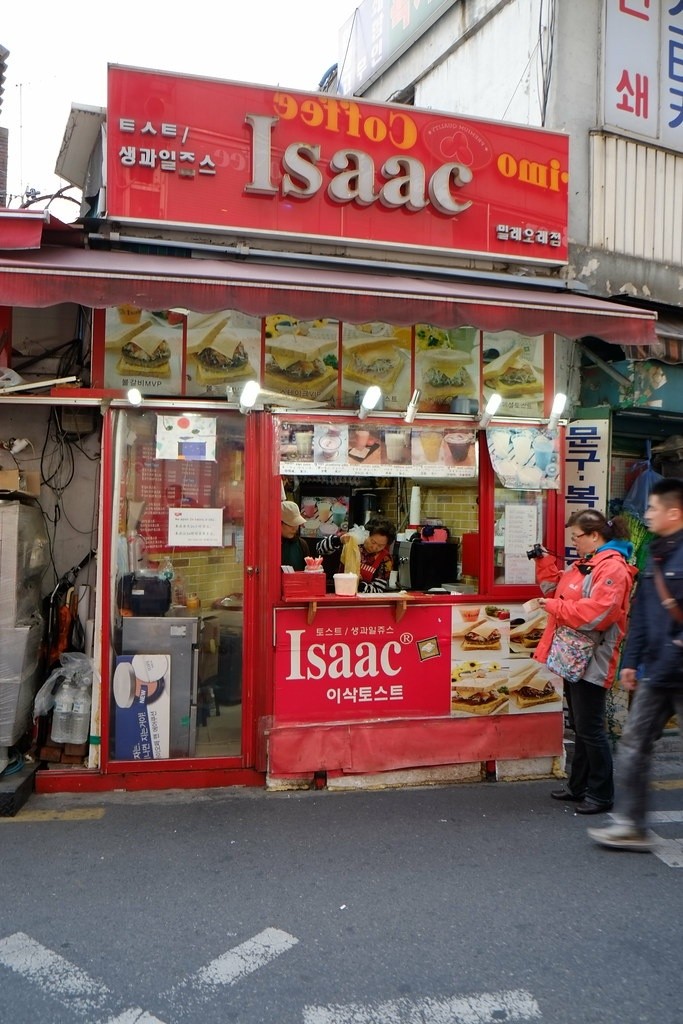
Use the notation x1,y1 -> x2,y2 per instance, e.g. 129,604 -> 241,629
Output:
127,388 -> 144,407
358,386 -> 381,419
404,389 -> 421,423
479,394 -> 502,427
239,380 -> 260,414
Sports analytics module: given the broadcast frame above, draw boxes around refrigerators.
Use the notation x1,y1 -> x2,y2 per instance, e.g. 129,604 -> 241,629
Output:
119,607 -> 198,757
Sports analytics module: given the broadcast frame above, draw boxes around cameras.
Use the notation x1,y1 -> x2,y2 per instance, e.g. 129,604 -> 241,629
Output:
526,543 -> 543,561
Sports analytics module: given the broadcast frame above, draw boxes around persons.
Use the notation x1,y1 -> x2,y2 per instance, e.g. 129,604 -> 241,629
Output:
315,515 -> 396,595
535,508 -> 640,816
587,477 -> 681,852
281,500 -> 310,571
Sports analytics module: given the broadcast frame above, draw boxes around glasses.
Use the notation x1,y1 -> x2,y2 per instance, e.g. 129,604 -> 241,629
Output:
571,532 -> 591,542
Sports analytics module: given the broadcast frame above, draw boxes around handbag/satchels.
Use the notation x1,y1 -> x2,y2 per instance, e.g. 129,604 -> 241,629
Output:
547,625 -> 597,683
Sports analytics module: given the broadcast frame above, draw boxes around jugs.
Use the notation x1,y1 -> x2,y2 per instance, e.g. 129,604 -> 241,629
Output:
426,516 -> 442,526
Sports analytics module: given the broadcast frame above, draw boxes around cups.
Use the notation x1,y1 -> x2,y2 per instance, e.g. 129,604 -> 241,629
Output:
302,497 -> 316,517
355,430 -> 369,450
330,505 -> 346,526
292,431 -> 314,458
419,432 -> 443,462
117,304 -> 142,325
397,486 -> 422,541
511,434 -> 532,462
304,519 -> 320,537
445,434 -> 470,461
532,436 -> 554,471
316,500 -> 332,522
319,522 -> 338,536
520,337 -> 538,361
318,436 -> 342,459
447,327 -> 477,351
493,459 -> 515,482
490,427 -> 510,458
461,606 -> 482,624
385,428 -> 406,462
518,465 -> 543,489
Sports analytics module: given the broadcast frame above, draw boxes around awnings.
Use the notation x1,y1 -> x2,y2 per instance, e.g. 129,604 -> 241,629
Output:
0,208 -> 73,249
2,243 -> 659,350
619,318 -> 682,367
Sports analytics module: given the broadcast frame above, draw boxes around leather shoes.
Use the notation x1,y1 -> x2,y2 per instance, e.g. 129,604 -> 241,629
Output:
575,802 -> 614,814
550,789 -> 584,802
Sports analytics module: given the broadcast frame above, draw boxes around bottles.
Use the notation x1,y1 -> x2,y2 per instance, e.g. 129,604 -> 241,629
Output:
159,554 -> 176,604
70,686 -> 91,744
60,671 -> 92,692
50,682 -> 70,744
127,525 -> 147,573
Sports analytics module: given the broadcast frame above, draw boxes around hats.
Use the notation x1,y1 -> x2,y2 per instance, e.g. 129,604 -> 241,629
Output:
280,501 -> 308,526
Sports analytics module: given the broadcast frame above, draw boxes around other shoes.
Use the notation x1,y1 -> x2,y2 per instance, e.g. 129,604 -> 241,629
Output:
587,820 -> 650,851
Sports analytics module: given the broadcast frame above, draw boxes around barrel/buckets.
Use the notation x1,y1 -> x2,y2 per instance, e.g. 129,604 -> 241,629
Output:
214,625 -> 242,702
353,494 -> 382,525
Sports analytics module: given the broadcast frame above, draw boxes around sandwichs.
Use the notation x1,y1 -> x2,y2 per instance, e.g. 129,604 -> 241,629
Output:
104,314 -> 544,399
451,615 -> 562,715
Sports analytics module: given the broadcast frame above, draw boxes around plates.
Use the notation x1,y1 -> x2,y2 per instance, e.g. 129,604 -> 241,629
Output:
349,444 -> 380,461
